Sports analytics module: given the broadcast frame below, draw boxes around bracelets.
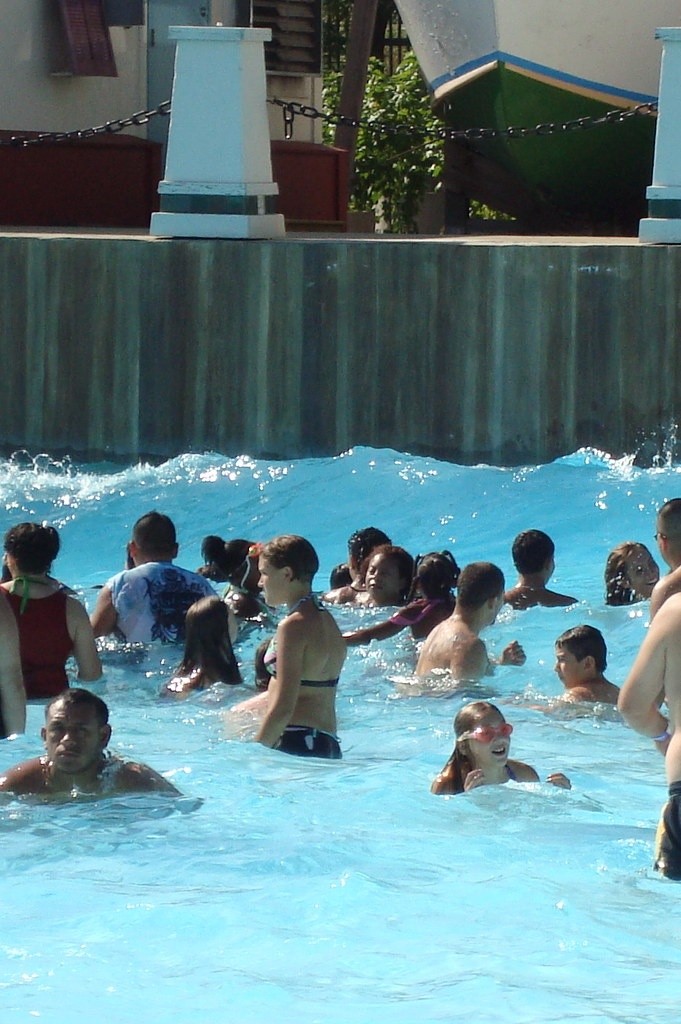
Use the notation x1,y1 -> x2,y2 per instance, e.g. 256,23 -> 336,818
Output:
651,721 -> 672,741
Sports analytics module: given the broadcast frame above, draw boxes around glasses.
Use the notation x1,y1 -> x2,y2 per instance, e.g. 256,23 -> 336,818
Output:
249,541 -> 264,558
457,723 -> 513,744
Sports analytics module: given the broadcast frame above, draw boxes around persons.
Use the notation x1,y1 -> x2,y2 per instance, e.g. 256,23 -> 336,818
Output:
431,702 -> 572,794
90,511 -> 273,690
553,623 -> 622,703
315,527 -> 461,646
604,542 -> 659,606
415,562 -> 528,680
0,688 -> 175,791
618,498 -> 681,880
253,535 -> 346,760
0,522 -> 101,735
504,530 -> 578,609
